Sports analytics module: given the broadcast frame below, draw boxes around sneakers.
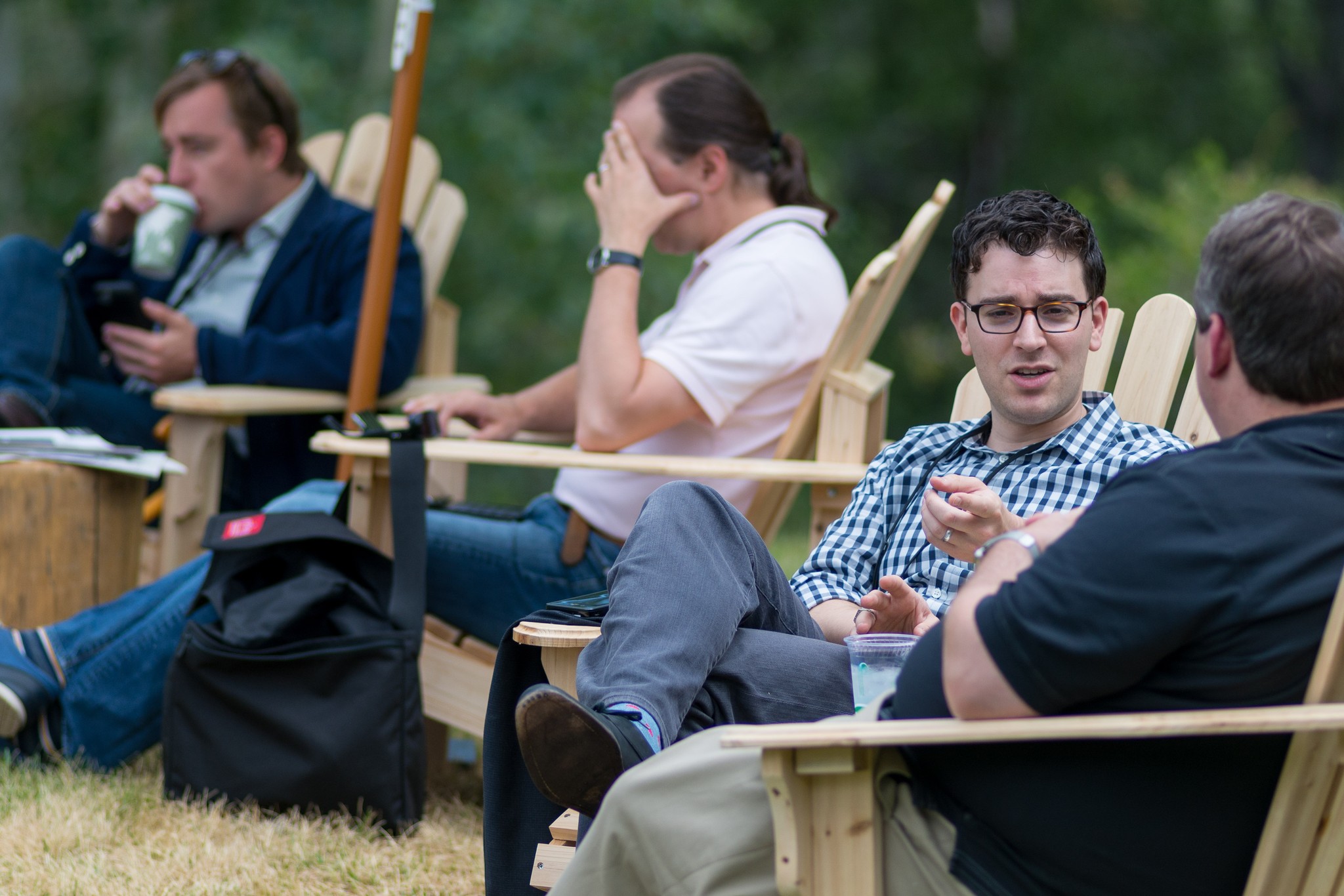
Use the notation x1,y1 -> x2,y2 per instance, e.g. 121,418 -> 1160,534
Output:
0,626 -> 58,739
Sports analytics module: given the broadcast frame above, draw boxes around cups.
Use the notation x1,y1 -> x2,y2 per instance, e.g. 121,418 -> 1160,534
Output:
844,633 -> 921,715
131,184 -> 200,280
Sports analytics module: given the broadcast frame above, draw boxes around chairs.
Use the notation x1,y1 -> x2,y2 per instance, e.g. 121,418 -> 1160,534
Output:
138,114 -> 1344,896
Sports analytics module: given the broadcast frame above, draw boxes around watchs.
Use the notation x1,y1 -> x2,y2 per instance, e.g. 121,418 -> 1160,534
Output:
584,247 -> 645,273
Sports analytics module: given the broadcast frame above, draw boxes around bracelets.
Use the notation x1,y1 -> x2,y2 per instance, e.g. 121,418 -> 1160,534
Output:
973,531 -> 1044,563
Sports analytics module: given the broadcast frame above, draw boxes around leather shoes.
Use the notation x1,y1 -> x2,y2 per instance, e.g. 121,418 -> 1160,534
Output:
515,684 -> 656,823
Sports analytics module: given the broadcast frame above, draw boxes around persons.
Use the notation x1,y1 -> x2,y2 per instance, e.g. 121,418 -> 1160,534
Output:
0,46 -> 428,530
513,190 -> 1195,851
0,53 -> 852,775
547,189 -> 1344,896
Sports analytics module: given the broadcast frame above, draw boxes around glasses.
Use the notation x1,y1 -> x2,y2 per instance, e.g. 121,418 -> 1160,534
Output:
960,298 -> 1093,334
179,47 -> 281,121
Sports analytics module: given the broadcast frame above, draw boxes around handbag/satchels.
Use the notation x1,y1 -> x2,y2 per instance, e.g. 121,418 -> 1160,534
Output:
162,407 -> 442,837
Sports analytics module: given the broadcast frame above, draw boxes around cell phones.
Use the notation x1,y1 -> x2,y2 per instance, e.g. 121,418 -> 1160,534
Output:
546,590 -> 609,618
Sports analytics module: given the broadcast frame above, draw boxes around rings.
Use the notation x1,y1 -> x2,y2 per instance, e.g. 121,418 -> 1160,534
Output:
595,163 -> 609,170
853,607 -> 877,624
941,527 -> 954,543
